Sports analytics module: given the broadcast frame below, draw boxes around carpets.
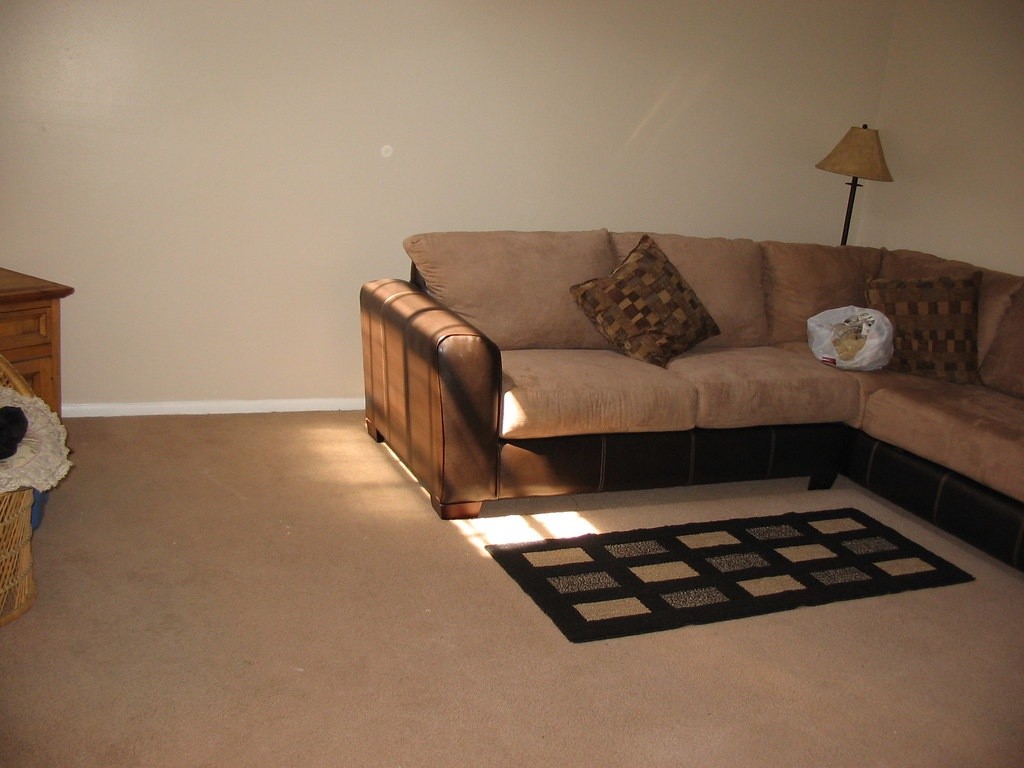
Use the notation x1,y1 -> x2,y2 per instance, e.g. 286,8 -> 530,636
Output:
485,506 -> 978,644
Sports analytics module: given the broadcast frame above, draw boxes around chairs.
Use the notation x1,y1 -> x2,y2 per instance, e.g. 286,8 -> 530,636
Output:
0,355 -> 72,627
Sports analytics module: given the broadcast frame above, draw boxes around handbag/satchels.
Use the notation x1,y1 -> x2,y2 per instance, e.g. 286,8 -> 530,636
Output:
806,305 -> 894,372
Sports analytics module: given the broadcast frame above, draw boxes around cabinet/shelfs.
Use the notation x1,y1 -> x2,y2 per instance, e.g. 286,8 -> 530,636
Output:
0,267 -> 74,422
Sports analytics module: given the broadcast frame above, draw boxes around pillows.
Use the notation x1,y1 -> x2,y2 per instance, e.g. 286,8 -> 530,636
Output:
570,235 -> 722,370
862,269 -> 984,384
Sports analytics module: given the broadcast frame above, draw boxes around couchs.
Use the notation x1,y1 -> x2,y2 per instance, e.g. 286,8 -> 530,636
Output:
360,230 -> 1024,572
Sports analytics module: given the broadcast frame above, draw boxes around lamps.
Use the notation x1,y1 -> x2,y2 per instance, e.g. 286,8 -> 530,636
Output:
814,125 -> 894,245
